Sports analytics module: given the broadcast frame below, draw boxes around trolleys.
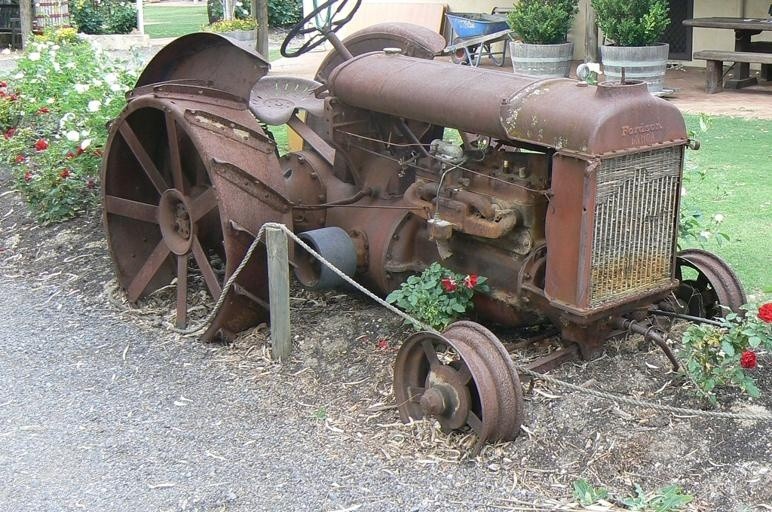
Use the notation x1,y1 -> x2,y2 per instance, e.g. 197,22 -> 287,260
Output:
435,11 -> 514,69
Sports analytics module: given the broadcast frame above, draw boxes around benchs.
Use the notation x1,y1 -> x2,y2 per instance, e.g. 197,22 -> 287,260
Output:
691,48 -> 772,94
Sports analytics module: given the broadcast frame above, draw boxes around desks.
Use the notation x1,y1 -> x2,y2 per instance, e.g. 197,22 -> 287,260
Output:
678,15 -> 772,93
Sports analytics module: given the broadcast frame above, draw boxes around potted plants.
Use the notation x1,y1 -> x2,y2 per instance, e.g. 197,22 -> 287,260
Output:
506,0 -> 581,81
589,0 -> 676,99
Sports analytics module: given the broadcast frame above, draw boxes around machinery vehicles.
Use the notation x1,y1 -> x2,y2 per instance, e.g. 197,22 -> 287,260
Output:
104,1 -> 746,457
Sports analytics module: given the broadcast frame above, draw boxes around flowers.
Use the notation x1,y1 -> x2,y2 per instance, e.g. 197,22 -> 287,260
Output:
209,15 -> 260,33
